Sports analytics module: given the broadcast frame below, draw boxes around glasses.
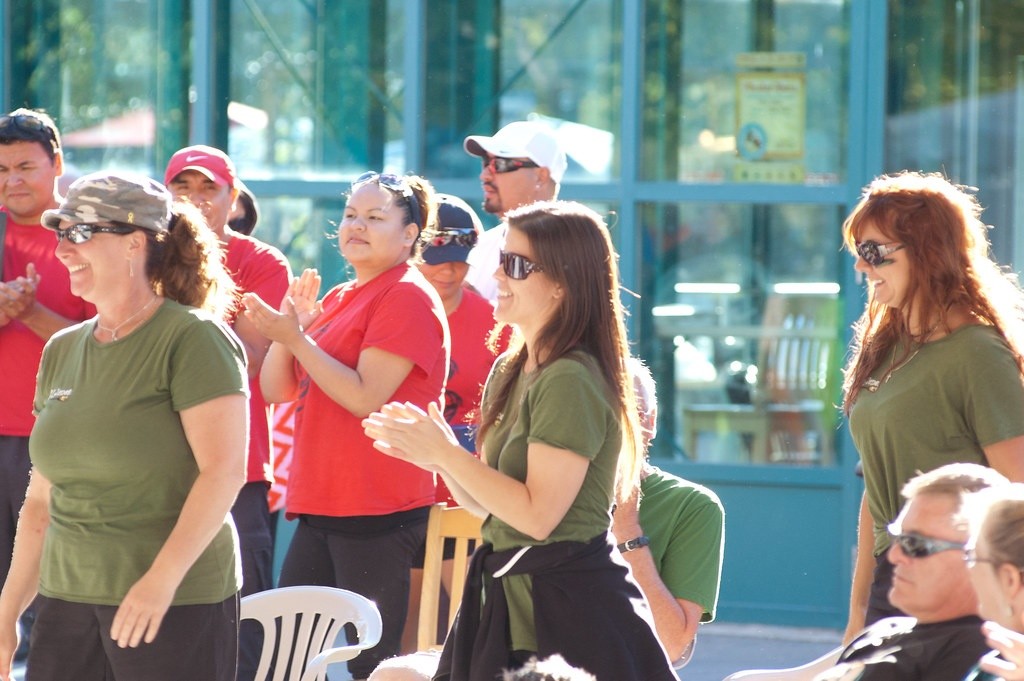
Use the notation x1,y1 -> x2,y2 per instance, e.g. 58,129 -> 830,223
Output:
963,550 -> 997,567
55,224 -> 136,244
500,248 -> 545,281
480,154 -> 539,174
352,171 -> 420,229
0,114 -> 53,138
885,523 -> 963,559
854,237 -> 907,265
227,217 -> 251,231
424,226 -> 479,249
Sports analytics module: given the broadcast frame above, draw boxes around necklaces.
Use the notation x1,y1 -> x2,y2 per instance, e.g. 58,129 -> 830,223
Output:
885,307 -> 958,384
92,294 -> 161,341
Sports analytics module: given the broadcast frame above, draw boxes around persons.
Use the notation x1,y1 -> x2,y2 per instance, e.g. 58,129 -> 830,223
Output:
163,121 -> 567,681
0,108 -> 251,681
835,168 -> 1024,645
723,464 -> 1013,681
358,202 -> 726,681
952,481 -> 1024,681
243,169 -> 452,681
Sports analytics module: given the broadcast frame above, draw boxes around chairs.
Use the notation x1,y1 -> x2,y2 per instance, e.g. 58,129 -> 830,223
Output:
417,501 -> 484,651
683,282 -> 839,466
240,586 -> 382,681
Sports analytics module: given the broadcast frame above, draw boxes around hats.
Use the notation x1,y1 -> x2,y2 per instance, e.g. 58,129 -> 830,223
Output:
164,144 -> 238,188
464,121 -> 567,185
41,169 -> 173,232
421,194 -> 482,265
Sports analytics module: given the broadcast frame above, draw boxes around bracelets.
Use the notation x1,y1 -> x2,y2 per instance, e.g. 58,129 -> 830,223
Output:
615,535 -> 651,553
